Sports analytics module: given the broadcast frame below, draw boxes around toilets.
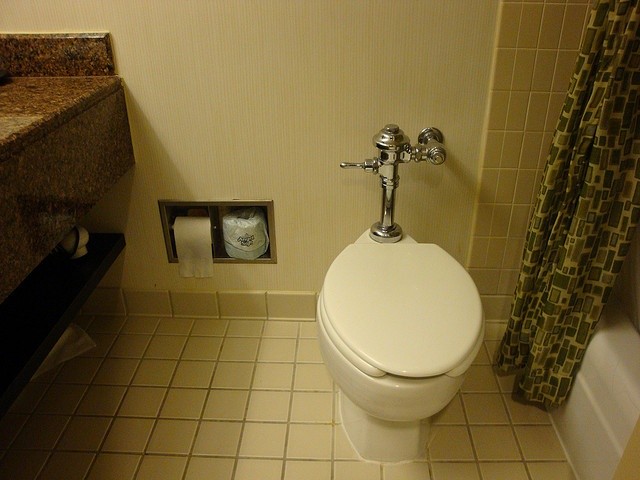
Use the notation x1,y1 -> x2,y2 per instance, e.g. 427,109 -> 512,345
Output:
316,125 -> 486,465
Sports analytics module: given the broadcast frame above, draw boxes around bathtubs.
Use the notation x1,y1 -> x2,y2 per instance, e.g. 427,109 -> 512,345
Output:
543,254 -> 639,467
